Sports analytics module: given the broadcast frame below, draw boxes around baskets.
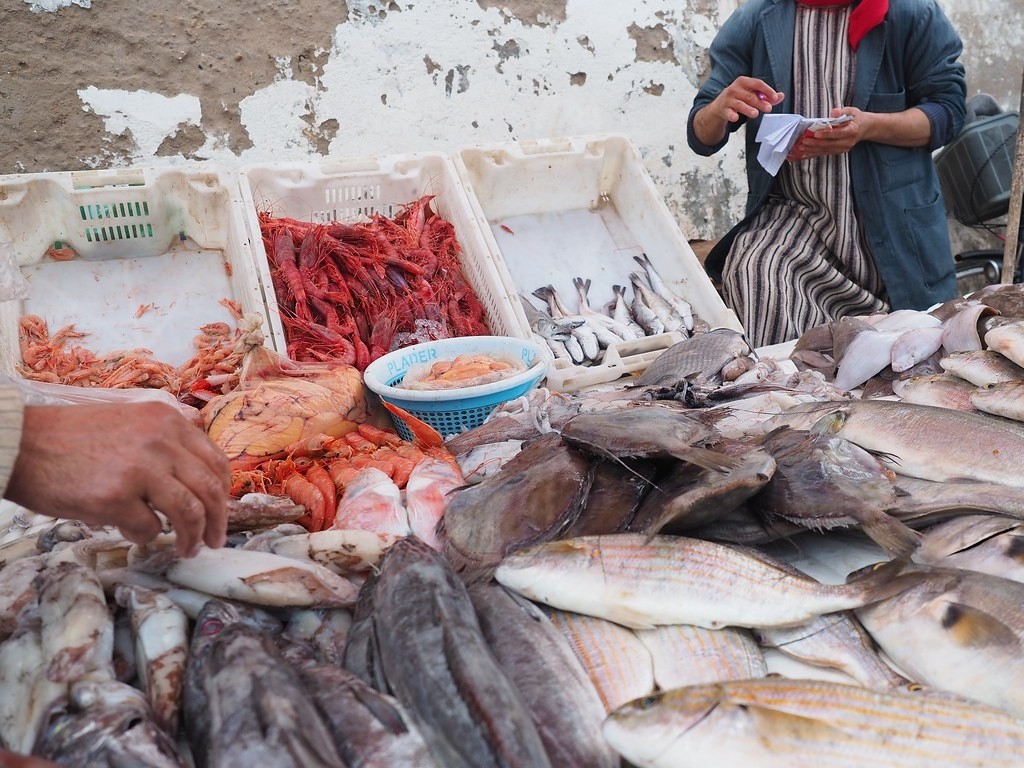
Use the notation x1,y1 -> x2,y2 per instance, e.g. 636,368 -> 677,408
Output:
363,334 -> 552,444
934,111 -> 1020,227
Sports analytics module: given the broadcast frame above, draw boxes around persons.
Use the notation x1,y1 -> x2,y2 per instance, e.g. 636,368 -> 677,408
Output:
1,377 -> 234,554
686,1 -> 970,348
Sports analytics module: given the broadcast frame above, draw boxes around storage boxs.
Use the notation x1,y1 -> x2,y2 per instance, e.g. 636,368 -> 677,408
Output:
361,333 -> 551,446
237,150 -> 528,388
0,161 -> 276,401
451,131 -> 750,399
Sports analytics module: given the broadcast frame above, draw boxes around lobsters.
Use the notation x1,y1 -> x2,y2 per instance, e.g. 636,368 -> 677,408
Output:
253,179 -> 491,370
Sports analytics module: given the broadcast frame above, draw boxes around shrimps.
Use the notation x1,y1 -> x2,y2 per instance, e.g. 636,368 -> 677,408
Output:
47,249 -> 75,261
13,298 -> 244,411
226,400 -> 444,533
416,353 -> 512,389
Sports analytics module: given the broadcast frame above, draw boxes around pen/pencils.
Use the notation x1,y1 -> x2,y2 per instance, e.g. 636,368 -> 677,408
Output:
755,91 -> 764,100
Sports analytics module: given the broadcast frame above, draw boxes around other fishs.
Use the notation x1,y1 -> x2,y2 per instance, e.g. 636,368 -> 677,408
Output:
434,280 -> 1024,589
516,253 -> 712,368
0,453 -> 1024,768
